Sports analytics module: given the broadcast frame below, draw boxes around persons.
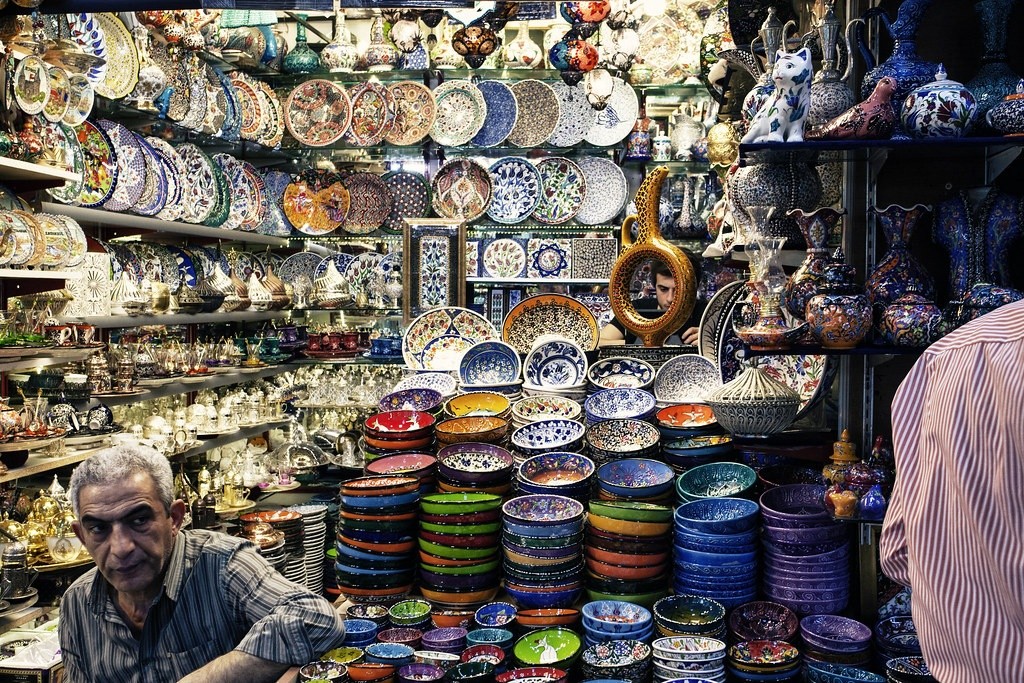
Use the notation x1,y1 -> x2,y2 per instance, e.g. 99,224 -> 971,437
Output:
598,247 -> 712,347
57,444 -> 347,683
879,296 -> 1024,683
427,34 -> 438,69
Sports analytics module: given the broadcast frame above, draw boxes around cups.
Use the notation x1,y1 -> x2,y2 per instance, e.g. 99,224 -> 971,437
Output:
3,317 -> 294,619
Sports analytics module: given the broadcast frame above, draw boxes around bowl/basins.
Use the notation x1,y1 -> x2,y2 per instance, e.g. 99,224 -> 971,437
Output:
298,294 -> 941,683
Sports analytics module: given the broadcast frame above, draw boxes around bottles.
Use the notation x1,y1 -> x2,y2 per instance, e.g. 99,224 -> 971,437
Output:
646,4 -> 1023,351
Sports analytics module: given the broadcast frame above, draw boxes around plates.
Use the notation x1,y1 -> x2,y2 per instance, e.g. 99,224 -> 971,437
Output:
234,504 -> 326,600
3,13 -> 642,310
